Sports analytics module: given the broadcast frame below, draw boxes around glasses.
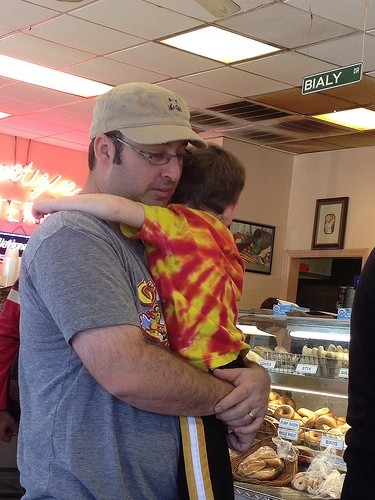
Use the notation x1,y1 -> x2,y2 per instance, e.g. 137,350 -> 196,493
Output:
114,138 -> 195,166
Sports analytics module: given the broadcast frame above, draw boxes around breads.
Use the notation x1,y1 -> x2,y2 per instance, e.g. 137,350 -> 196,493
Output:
235,445 -> 285,480
250,336 -> 354,381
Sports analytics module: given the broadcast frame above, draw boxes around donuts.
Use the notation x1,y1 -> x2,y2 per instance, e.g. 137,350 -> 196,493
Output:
263,390 -> 350,455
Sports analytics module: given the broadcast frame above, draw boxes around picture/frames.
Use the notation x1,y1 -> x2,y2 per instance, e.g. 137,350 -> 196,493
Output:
310,197 -> 349,250
226,218 -> 276,276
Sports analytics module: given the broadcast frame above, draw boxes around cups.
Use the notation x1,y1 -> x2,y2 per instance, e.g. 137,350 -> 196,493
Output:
1,247 -> 19,287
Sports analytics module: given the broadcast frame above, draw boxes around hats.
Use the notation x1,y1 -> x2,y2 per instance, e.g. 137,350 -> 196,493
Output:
89,82 -> 208,149
